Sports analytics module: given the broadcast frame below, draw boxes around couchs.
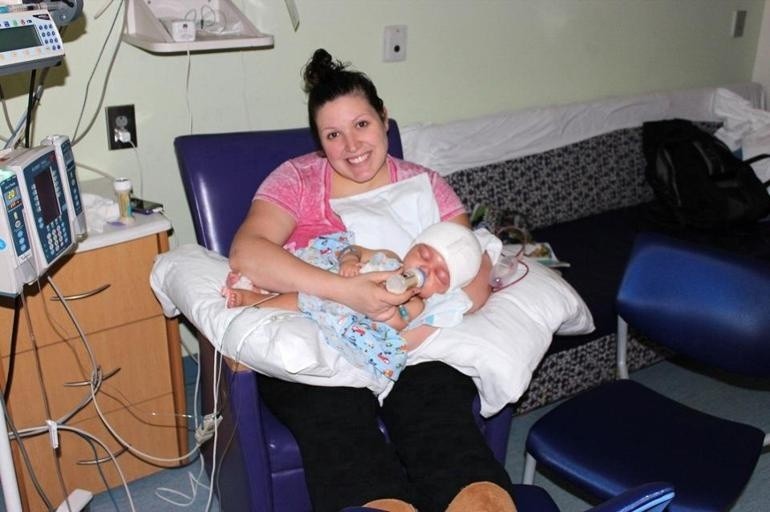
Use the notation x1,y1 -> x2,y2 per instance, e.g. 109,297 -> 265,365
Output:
399,78 -> 768,416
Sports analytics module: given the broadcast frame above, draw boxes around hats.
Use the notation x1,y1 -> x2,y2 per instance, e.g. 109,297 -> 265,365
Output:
414,222 -> 482,295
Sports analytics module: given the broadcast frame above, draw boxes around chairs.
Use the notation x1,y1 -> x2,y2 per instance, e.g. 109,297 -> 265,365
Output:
339,473 -> 676,512
519,234 -> 769,510
176,116 -> 516,512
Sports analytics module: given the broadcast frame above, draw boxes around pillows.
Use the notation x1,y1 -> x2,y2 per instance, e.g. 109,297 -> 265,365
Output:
329,172 -> 442,257
408,258 -> 596,416
149,238 -> 383,393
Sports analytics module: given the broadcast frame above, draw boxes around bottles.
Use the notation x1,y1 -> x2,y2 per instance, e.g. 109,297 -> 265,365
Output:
113,176 -> 133,219
387,266 -> 427,294
484,250 -> 519,289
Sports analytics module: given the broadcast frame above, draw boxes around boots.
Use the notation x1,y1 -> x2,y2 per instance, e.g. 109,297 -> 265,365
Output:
339,498 -> 418,512
443,480 -> 520,512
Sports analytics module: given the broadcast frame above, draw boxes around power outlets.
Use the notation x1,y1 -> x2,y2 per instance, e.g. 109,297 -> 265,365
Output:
103,103 -> 140,150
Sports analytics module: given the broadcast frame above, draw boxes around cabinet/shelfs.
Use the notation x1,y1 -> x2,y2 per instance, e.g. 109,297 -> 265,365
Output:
0,216 -> 194,512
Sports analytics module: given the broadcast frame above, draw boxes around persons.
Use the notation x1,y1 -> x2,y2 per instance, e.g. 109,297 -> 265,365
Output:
229,49 -> 518,512
226,221 -> 482,332
